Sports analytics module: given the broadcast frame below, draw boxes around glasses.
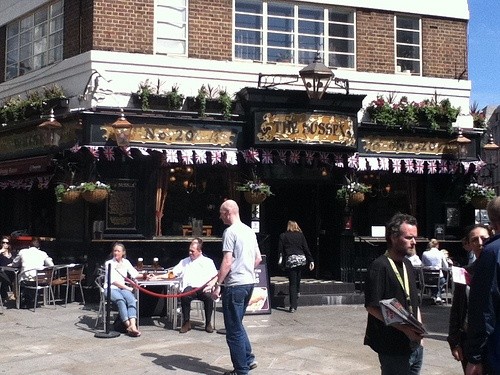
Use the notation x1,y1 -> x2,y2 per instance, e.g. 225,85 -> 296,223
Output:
2,241 -> 10,245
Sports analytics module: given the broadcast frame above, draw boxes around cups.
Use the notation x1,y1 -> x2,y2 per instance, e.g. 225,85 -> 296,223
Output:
168,270 -> 174,279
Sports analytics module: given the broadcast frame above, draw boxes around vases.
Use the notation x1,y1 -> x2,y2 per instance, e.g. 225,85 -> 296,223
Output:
63,190 -> 81,204
82,188 -> 109,203
471,198 -> 491,208
350,191 -> 365,203
244,191 -> 266,205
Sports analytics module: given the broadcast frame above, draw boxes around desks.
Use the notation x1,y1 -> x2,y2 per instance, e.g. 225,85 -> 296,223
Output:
413,265 -> 455,305
181,225 -> 213,236
0,265 -> 62,309
132,265 -> 179,330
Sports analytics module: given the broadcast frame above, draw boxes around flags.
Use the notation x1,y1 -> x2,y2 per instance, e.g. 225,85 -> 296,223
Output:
0,175 -> 53,190
71,143 -> 150,160
152,149 -> 359,169
357,157 -> 485,173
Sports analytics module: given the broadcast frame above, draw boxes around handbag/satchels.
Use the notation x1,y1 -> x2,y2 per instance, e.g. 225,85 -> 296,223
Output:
277,233 -> 285,270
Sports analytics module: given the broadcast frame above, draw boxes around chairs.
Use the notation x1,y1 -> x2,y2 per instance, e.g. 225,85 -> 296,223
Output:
419,266 -> 450,305
91,266 -> 221,331
17,263 -> 85,312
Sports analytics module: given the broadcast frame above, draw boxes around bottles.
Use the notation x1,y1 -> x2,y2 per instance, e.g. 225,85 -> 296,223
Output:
153,258 -> 159,271
137,257 -> 143,271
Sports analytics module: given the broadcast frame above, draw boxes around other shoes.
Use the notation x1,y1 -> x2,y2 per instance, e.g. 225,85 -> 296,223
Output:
296,292 -> 300,297
179,322 -> 192,333
205,322 -> 214,333
127,326 -> 142,337
289,308 -> 296,313
224,370 -> 249,375
435,297 -> 446,305
248,361 -> 258,370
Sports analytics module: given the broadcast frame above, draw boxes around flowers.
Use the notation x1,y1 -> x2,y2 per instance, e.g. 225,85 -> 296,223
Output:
338,180 -> 371,203
55,180 -> 115,203
461,181 -> 497,205
371,91 -> 433,130
234,176 -> 273,200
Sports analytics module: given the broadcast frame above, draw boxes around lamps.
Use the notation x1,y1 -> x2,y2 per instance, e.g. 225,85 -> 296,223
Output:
259,45 -> 350,108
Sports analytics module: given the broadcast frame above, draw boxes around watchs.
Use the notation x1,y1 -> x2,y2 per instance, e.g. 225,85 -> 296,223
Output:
215,282 -> 218,285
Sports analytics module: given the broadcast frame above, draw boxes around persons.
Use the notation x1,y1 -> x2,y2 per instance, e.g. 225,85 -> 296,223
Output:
405,245 -> 422,266
462,237 -> 477,265
464,196 -> 500,375
422,238 -> 449,304
440,249 -> 453,267
152,238 -> 218,333
447,224 -> 495,375
0,236 -> 16,304
211,200 -> 262,375
104,242 -> 150,336
363,213 -> 424,375
13,237 -> 54,308
279,220 -> 314,313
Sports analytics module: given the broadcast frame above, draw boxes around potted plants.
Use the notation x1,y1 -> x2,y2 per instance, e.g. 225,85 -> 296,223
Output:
0,84 -> 69,126
185,84 -> 237,115
131,79 -> 183,110
